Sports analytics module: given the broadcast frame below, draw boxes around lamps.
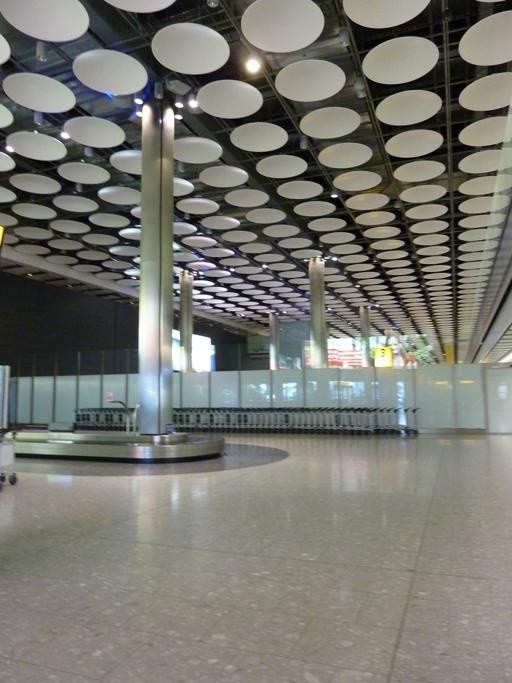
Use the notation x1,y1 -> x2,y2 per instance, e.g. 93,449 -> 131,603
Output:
133,80 -> 198,121
